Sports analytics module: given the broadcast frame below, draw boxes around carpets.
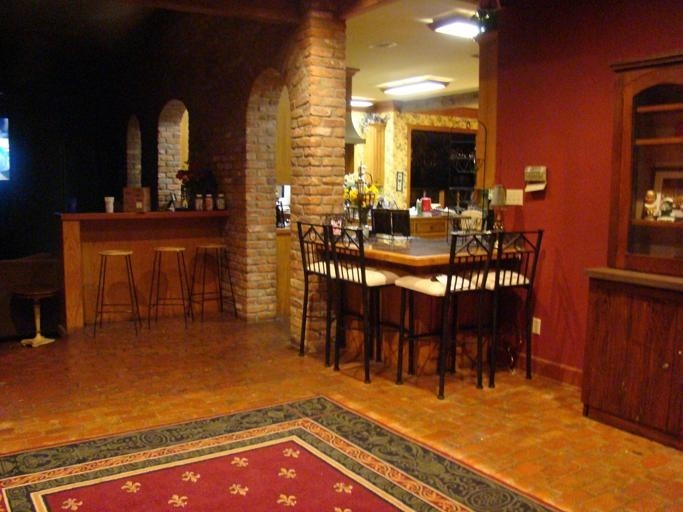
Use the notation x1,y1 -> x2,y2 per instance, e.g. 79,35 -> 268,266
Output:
0,392 -> 566,512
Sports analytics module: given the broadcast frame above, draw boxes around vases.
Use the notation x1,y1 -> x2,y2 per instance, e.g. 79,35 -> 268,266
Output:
357,206 -> 370,226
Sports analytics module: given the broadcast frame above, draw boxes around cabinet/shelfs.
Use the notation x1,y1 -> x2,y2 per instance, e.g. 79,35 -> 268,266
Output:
581,268 -> 683,452
579,51 -> 683,276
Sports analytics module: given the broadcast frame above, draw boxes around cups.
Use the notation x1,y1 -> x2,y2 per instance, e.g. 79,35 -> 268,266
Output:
104,196 -> 115,214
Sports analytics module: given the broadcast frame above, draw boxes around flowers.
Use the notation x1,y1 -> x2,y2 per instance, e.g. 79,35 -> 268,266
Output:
343,170 -> 377,215
176,159 -> 213,186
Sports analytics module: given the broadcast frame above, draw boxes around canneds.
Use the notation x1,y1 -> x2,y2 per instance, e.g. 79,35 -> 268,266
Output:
205,194 -> 215,210
216,193 -> 226,209
195,194 -> 204,211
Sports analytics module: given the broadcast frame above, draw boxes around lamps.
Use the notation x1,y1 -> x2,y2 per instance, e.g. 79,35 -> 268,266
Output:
426,9 -> 484,41
376,75 -> 452,96
350,97 -> 374,110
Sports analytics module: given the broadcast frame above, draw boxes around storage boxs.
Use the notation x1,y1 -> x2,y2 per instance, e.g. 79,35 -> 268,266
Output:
122,186 -> 151,214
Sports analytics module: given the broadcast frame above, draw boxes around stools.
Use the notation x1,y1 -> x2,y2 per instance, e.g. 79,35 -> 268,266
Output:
91,244 -> 236,338
10,278 -> 62,348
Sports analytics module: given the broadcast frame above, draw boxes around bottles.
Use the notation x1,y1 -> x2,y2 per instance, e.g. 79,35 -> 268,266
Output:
181,191 -> 226,212
